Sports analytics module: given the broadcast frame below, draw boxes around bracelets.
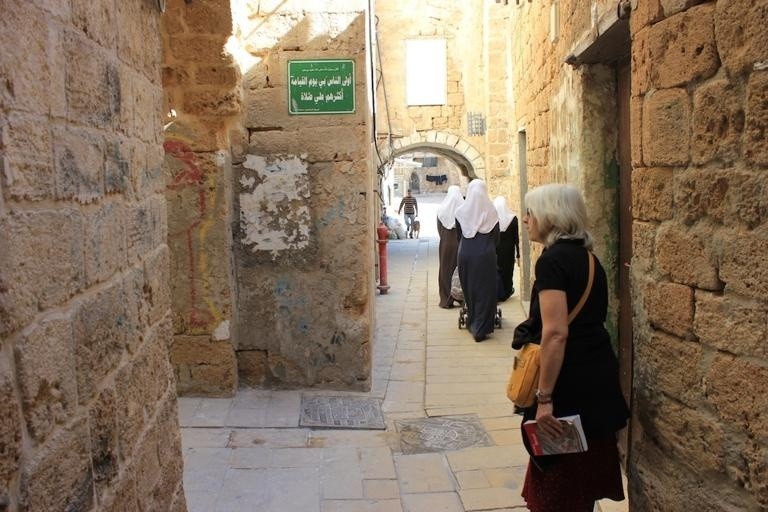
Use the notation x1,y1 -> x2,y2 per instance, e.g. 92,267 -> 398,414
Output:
535,387 -> 552,399
537,396 -> 552,404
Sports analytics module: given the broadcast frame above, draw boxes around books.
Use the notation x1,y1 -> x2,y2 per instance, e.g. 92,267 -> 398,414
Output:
523,414 -> 588,456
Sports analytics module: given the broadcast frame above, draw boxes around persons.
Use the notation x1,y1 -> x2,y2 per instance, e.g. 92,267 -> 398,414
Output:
435,184 -> 466,308
492,196 -> 522,303
510,183 -> 635,511
398,189 -> 419,239
453,178 -> 502,341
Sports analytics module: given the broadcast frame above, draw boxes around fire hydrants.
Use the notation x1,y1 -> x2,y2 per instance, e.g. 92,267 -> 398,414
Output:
374,220 -> 391,295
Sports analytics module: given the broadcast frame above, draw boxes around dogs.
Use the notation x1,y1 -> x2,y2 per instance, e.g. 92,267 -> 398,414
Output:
413,220 -> 420,239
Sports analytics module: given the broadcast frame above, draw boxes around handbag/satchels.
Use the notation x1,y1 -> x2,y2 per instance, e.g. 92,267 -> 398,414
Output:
507,341 -> 541,408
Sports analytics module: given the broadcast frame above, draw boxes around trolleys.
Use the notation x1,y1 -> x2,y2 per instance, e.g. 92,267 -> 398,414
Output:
459,299 -> 503,328
